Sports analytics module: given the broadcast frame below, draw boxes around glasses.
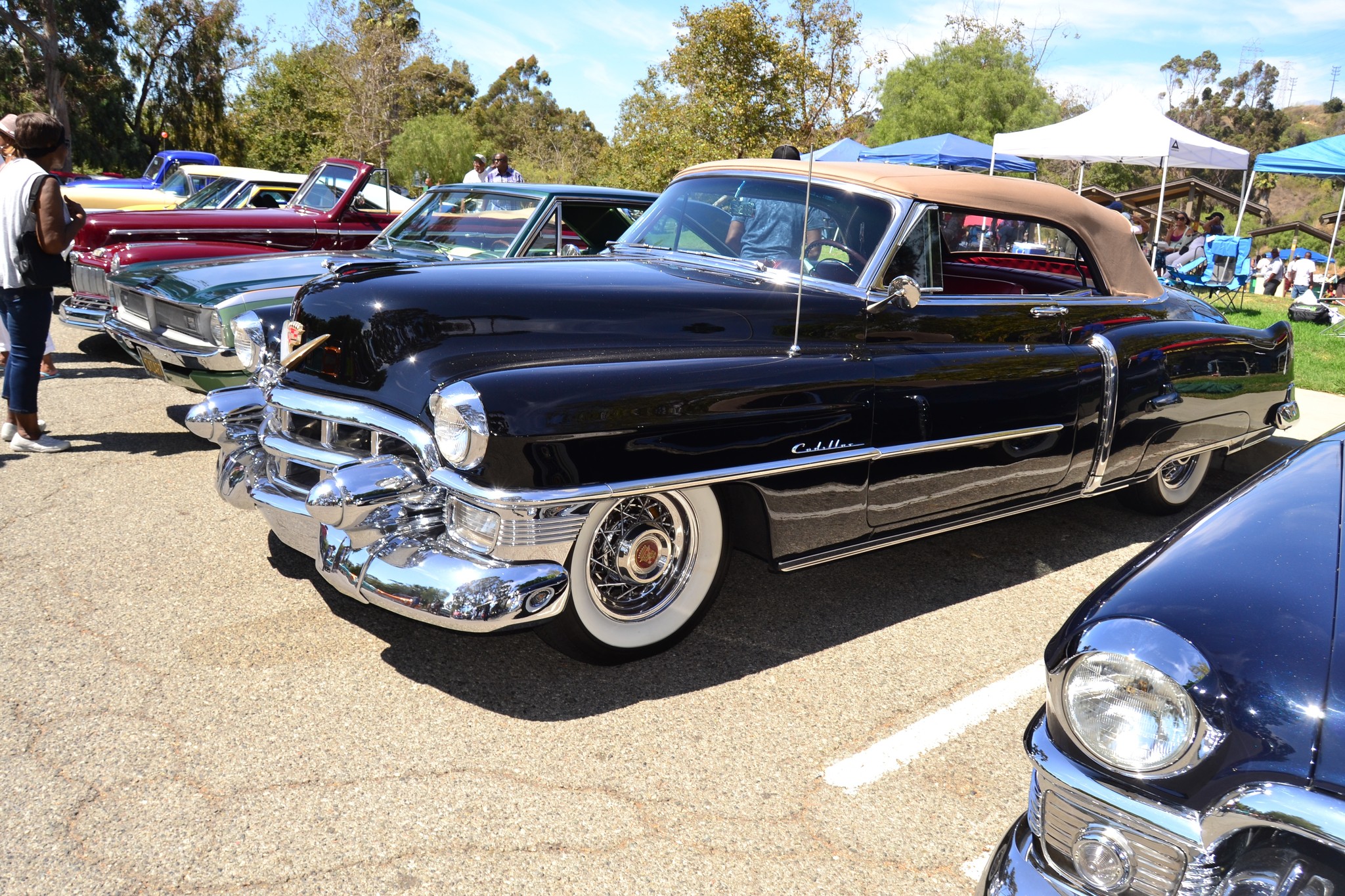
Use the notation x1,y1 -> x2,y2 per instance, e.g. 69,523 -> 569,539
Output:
1174,216 -> 1185,222
63,139 -> 70,149
493,158 -> 502,162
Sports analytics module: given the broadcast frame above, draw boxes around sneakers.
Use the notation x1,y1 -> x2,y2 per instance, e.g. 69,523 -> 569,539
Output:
10,432 -> 71,453
1,419 -> 47,440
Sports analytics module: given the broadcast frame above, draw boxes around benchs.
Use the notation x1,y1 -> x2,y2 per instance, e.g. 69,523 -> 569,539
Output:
933,273 -> 1030,294
933,262 -> 1111,296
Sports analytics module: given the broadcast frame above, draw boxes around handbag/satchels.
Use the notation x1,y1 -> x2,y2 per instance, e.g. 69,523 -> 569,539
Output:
1287,302 -> 1332,326
1179,246 -> 1189,255
14,174 -> 68,290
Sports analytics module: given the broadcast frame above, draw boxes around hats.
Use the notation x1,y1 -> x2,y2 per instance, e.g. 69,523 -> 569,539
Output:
1270,248 -> 1280,254
1205,212 -> 1225,221
771,145 -> 800,160
1177,212 -> 1191,225
1294,255 -> 1300,259
1121,212 -> 1131,220
1105,201 -> 1123,210
472,154 -> 486,164
0,113 -> 19,140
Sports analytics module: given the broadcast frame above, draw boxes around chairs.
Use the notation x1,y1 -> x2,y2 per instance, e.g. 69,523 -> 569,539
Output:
962,222 -> 1029,252
1166,234 -> 1253,316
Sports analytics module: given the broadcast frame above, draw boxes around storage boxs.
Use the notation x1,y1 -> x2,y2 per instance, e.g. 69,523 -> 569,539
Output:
1012,242 -> 1046,256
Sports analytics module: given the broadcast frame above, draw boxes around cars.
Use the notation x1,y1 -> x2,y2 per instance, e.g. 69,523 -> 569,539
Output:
184,161 -> 1301,667
45,150 -> 468,262
975,425 -> 1344,896
104,181 -> 746,397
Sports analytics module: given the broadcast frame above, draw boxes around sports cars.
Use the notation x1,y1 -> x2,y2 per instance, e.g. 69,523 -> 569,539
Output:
59,155 -> 587,332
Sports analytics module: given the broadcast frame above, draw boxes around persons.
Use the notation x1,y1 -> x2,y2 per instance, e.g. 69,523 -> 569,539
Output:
481,153 -> 529,210
486,153 -> 497,211
1154,212 -> 1194,277
1162,212 -> 1225,279
423,178 -> 442,213
0,111 -> 86,453
724,145 -> 826,261
1105,201 -> 1150,235
1283,256 -> 1300,297
1291,252 -> 1316,298
1263,248 -> 1283,295
1322,284 -> 1337,304
0,114 -> 61,381
459,153 -> 490,214
1244,255 -> 1261,293
996,220 -> 1025,252
1257,254 -> 1270,273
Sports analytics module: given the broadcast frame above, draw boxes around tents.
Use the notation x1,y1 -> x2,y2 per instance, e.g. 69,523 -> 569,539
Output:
1215,135 -> 1345,300
800,136 -> 872,253
856,133 -> 1040,252
978,92 -> 1250,274
1264,247 -> 1338,284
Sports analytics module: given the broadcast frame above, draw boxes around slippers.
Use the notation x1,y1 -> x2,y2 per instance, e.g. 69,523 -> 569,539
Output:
38,372 -> 60,381
0,366 -> 6,369
